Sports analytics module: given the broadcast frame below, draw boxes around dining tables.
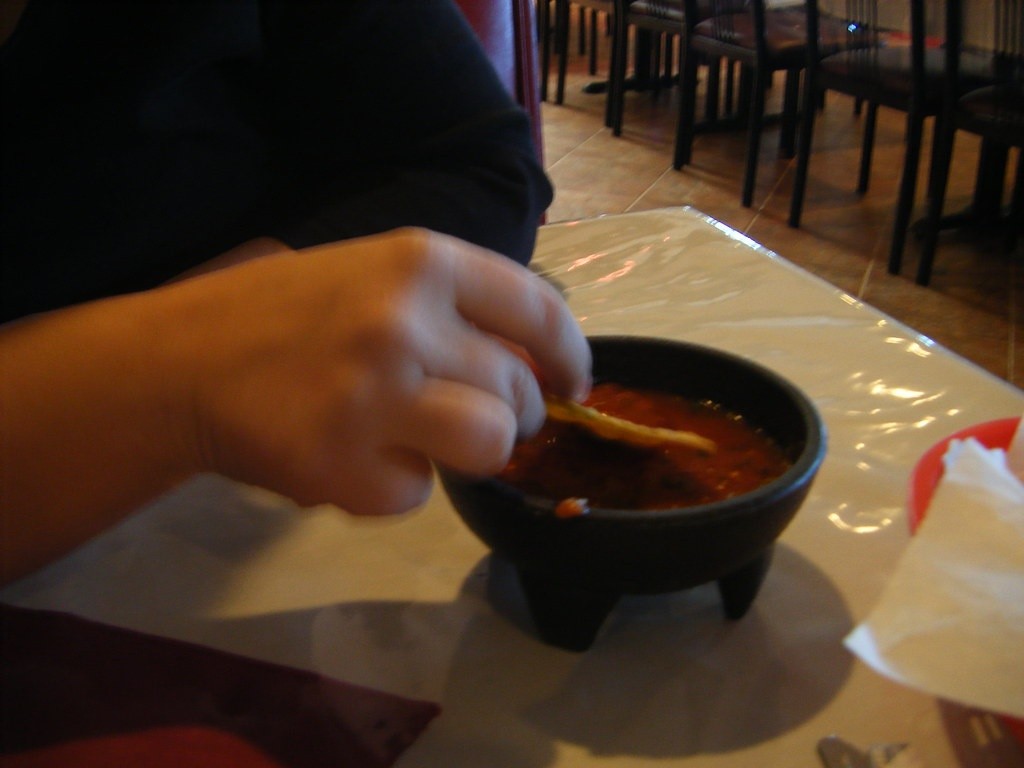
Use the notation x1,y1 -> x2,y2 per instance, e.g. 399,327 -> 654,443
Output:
0,207 -> 1024,768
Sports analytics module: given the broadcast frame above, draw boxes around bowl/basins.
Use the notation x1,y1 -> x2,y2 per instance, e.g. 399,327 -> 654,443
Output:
910,416 -> 1021,540
435,328 -> 823,591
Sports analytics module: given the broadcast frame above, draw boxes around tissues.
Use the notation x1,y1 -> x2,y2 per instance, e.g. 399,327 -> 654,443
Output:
835,434 -> 1023,767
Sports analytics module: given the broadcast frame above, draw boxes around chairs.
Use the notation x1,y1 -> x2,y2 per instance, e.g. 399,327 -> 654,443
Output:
533,0 -> 1024,286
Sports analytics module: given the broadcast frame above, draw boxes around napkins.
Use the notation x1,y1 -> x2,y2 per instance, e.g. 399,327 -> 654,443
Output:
840,435 -> 1024,718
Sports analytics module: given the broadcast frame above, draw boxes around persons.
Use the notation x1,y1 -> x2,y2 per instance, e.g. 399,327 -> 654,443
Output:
0,0 -> 592,591
